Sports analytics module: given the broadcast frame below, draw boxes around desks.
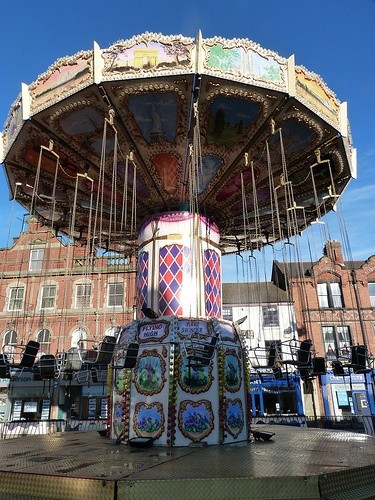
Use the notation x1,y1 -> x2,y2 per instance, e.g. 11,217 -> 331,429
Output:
79,419 -> 107,431
5,419 -> 66,442
250,413 -> 308,428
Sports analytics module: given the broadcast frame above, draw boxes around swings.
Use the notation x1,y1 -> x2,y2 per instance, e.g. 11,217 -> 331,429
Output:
0,100 -> 374,388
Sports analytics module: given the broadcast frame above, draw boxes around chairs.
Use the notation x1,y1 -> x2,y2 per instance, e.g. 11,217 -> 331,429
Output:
276,339 -> 311,366
83,351 -> 98,369
63,348 -> 84,372
96,337 -> 117,368
258,371 -> 270,383
184,333 -> 217,367
37,355 -> 58,382
272,367 -> 290,380
332,360 -> 349,376
115,343 -> 138,369
0,353 -> 12,379
303,358 -> 325,377
14,341 -> 39,372
247,343 -> 275,368
352,345 -> 372,373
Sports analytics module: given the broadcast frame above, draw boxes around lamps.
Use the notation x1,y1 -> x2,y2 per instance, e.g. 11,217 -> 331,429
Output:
129,436 -> 151,447
254,429 -> 274,439
